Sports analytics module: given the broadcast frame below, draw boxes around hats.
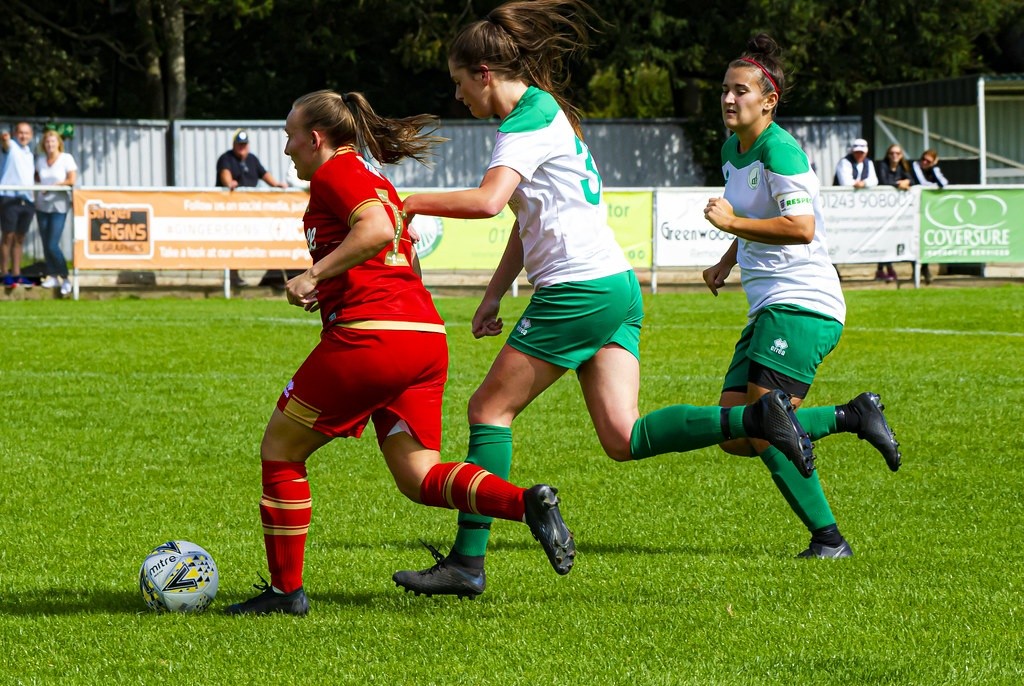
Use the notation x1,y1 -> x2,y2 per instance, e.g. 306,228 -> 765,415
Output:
234,130 -> 248,144
853,139 -> 868,153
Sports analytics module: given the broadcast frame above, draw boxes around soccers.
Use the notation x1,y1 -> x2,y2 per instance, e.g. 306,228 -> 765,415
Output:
139,539 -> 220,614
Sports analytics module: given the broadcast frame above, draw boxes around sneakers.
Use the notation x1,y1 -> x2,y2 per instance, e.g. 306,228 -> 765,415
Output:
224,585 -> 310,618
752,389 -> 818,478
524,484 -> 576,575
392,556 -> 485,601
850,392 -> 902,472
794,535 -> 853,559
4,274 -> 34,288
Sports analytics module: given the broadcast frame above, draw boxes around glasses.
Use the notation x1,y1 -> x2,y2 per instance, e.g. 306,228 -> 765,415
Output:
922,157 -> 933,164
888,152 -> 901,155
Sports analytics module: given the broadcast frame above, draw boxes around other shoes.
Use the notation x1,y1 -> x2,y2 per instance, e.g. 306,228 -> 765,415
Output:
875,270 -> 897,281
923,272 -> 933,284
232,278 -> 248,287
42,278 -> 58,287
60,278 -> 72,294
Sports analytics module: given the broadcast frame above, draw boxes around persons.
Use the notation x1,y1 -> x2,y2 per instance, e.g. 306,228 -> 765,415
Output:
34,131 -> 78,296
391,3 -> 817,600
702,35 -> 902,559
832,137 -> 878,189
874,144 -> 915,282
909,149 -> 948,284
223,90 -> 579,617
0,121 -> 36,288
217,130 -> 288,288
